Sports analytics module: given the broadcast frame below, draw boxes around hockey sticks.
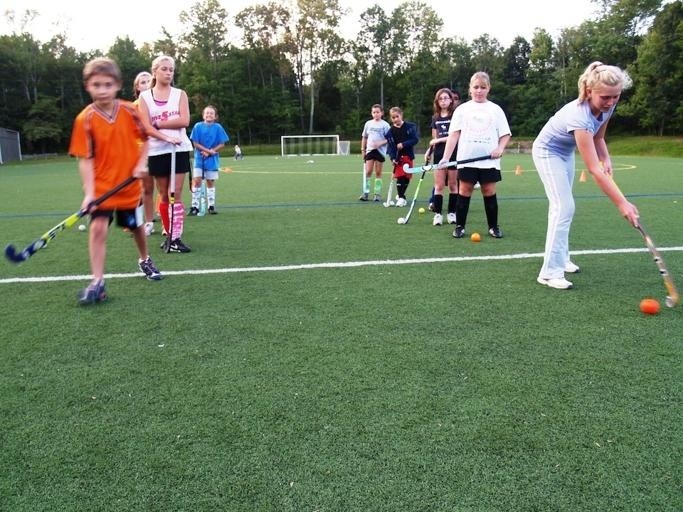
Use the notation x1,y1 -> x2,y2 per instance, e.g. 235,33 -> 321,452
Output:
401,155 -> 510,174
382,146 -> 400,207
397,143 -> 435,224
163,143 -> 176,254
362,157 -> 372,194
197,154 -> 208,216
633,221 -> 679,309
4,176 -> 140,263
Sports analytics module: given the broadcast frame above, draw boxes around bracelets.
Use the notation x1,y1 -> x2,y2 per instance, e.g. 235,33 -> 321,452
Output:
360,147 -> 366,152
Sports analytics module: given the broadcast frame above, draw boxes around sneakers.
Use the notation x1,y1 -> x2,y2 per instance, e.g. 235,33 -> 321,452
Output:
78,206 -> 217,305
359,193 -> 579,290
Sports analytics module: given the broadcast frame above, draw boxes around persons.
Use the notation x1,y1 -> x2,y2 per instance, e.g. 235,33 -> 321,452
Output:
528,61 -> 642,291
357,103 -> 390,204
130,70 -> 168,237
234,144 -> 243,162
65,55 -> 166,309
189,104 -> 231,217
424,87 -> 468,228
138,53 -> 196,254
438,70 -> 513,239
428,90 -> 463,212
384,105 -> 420,208
